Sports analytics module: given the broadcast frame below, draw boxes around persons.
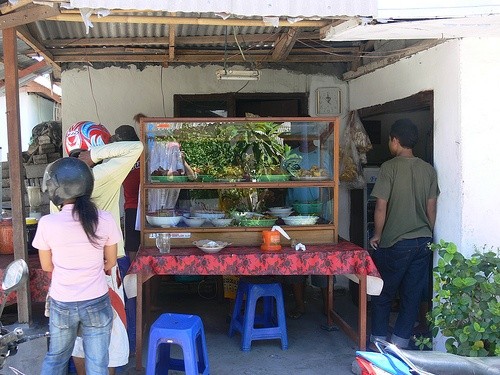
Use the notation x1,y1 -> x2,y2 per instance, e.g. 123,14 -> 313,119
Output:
280,114 -> 336,319
367,119 -> 442,353
49,119 -> 266,375
32,157 -> 123,375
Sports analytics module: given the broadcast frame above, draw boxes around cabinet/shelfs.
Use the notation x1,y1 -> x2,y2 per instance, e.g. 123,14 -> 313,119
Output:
138,116 -> 341,246
363,168 -> 382,248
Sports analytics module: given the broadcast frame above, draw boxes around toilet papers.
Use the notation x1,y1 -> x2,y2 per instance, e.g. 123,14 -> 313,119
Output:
261,226 -> 291,250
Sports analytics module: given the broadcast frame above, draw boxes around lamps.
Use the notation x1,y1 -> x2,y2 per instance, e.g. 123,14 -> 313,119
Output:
215,69 -> 261,82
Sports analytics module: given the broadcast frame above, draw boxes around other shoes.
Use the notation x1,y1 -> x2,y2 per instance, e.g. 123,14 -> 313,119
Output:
369,342 -> 384,352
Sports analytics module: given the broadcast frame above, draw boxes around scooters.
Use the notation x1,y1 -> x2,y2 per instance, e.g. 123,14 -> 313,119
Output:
0,258 -> 53,375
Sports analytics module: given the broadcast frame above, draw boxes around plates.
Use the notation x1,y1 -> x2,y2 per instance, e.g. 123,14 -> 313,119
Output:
150,174 -> 331,182
238,219 -> 277,227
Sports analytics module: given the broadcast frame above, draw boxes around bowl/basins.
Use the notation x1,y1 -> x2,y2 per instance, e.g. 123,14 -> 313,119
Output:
145,201 -> 324,228
199,246 -> 224,253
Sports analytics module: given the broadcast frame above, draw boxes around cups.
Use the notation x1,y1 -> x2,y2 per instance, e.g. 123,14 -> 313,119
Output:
155,234 -> 171,254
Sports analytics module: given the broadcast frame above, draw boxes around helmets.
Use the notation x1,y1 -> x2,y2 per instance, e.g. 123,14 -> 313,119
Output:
65,121 -> 113,164
46,157 -> 94,206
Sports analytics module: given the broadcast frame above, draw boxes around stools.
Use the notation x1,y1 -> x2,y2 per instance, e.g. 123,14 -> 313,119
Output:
145,313 -> 210,375
228,278 -> 289,353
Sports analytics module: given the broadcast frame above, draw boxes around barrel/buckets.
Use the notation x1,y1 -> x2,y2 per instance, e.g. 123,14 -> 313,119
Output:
0,218 -> 14,254
25,217 -> 39,255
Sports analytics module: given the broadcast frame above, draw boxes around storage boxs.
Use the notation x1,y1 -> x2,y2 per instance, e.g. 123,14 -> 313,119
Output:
222,274 -> 247,301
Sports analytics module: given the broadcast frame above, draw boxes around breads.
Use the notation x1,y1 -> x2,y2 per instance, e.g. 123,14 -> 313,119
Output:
339,121 -> 372,181
296,165 -> 327,177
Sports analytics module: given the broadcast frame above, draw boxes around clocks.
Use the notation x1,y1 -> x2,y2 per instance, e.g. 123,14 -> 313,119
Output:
316,87 -> 343,115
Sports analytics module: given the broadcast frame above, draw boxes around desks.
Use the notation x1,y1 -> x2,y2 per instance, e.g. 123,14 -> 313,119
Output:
0,253 -> 52,305
127,240 -> 383,371
